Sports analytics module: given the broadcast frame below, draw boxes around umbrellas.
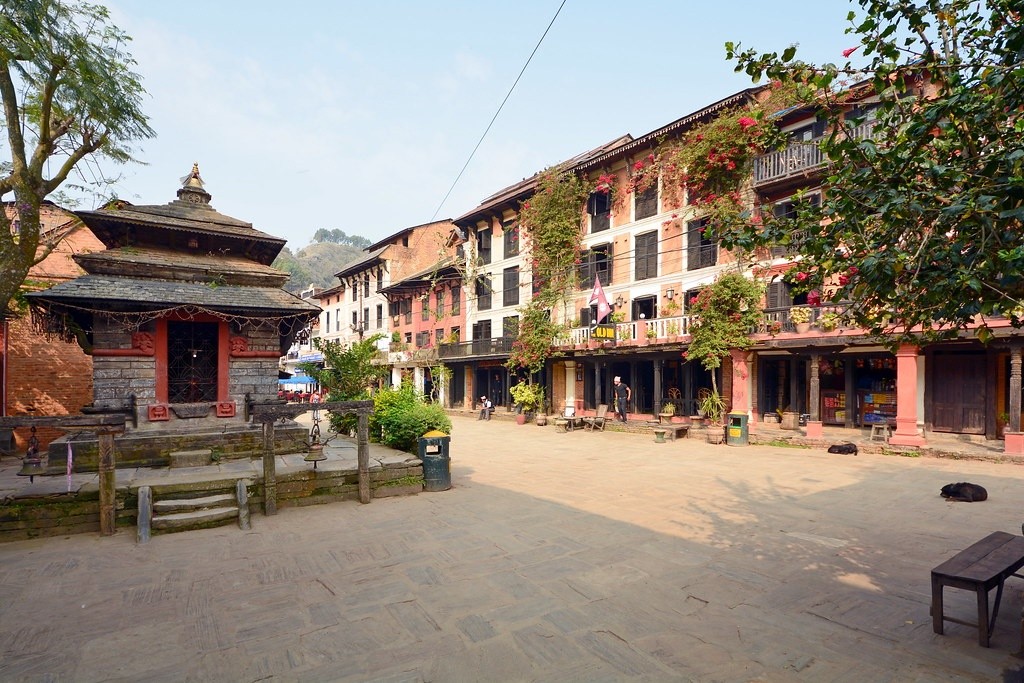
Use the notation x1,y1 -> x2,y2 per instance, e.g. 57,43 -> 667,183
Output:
279,376 -> 318,388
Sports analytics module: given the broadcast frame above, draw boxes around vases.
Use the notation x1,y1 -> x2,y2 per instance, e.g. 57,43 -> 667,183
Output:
794,323 -> 810,334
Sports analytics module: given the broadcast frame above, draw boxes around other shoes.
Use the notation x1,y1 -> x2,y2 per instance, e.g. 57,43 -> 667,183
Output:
312,417 -> 322,421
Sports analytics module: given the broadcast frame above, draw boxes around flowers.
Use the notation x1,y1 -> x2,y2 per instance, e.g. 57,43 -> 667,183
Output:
789,307 -> 811,324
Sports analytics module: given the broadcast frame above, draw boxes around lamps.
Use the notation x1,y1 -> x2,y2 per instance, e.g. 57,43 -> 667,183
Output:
666,288 -> 674,299
616,296 -> 623,308
188,345 -> 203,357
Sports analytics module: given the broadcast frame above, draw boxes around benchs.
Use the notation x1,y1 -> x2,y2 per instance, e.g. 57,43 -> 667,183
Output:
930,530 -> 1024,648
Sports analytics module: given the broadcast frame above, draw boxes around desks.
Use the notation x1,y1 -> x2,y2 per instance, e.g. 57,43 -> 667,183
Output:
561,415 -> 583,432
659,423 -> 690,441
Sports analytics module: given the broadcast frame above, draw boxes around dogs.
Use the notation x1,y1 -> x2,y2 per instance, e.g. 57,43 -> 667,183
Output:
940,482 -> 988,502
828,443 -> 858,456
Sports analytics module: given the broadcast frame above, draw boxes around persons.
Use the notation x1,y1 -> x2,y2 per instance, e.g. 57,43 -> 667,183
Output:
279,389 -> 282,391
492,374 -> 501,406
310,390 -> 322,421
614,376 -> 631,422
476,396 -> 495,419
290,389 -> 293,393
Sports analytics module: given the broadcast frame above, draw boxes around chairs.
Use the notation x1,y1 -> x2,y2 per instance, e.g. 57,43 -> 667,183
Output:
668,388 -> 684,413
582,404 -> 608,432
697,388 -> 711,411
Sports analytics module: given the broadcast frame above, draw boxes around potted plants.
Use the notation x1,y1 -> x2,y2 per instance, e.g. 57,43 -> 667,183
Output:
510,382 -> 533,424
534,388 -> 547,425
1000,412 -> 1010,435
699,394 -> 728,444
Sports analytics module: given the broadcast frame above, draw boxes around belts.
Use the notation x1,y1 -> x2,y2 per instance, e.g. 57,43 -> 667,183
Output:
619,397 -> 627,400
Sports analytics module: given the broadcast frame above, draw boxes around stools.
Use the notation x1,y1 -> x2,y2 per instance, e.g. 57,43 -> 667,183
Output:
870,423 -> 891,442
556,420 -> 569,432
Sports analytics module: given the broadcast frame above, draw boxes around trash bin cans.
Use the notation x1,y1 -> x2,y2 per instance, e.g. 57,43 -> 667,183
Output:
727,410 -> 749,446
420,430 -> 452,491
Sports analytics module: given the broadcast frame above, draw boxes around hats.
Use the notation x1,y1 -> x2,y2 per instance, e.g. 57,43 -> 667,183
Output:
481,395 -> 487,399
614,376 -> 620,381
